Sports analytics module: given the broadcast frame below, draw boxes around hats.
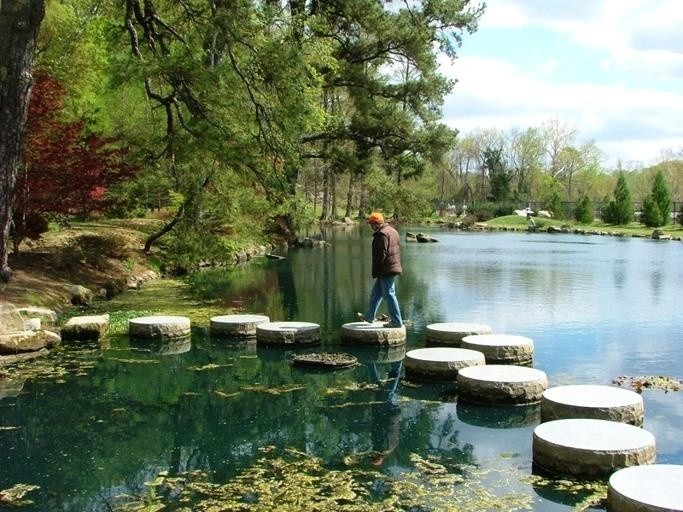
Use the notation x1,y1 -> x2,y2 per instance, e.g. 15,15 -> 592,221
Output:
369,212 -> 385,225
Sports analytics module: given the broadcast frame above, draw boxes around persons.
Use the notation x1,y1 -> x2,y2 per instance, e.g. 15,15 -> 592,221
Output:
357,212 -> 403,328
526,207 -> 535,225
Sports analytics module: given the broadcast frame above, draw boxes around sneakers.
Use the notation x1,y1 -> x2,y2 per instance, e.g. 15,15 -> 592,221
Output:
384,322 -> 401,328
358,312 -> 373,324
